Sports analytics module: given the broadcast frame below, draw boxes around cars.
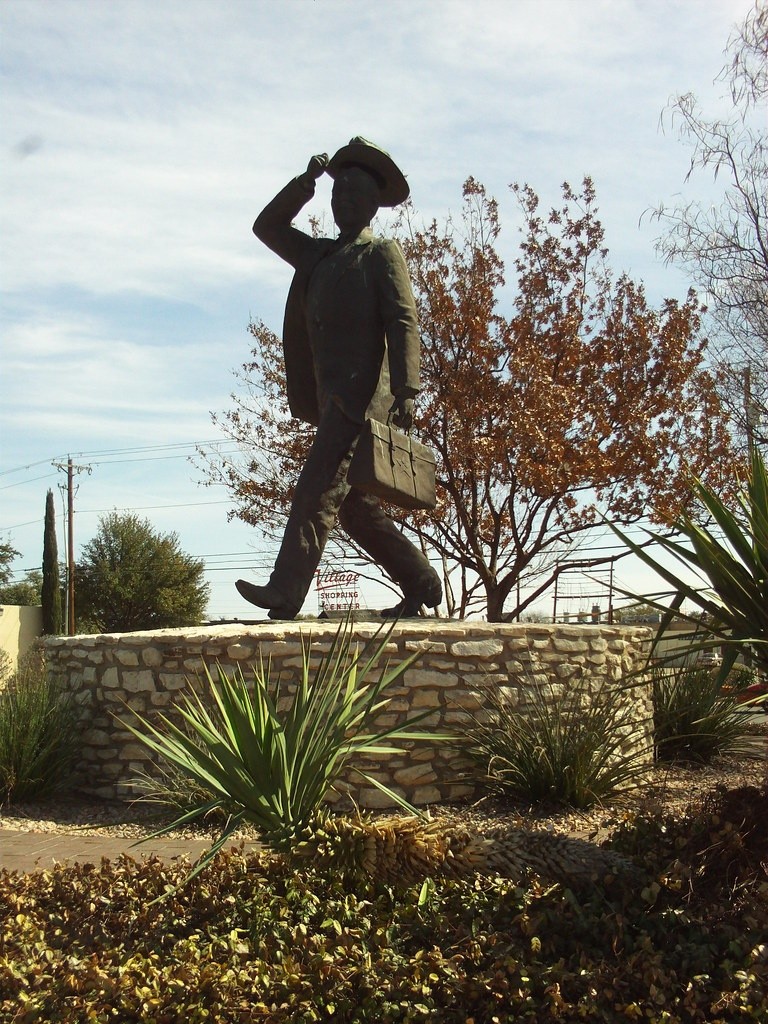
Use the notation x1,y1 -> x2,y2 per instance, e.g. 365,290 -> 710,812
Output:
695,652 -> 723,667
733,680 -> 768,714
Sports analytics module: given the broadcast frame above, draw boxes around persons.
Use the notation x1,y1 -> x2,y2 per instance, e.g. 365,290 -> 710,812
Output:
234,137 -> 443,618
